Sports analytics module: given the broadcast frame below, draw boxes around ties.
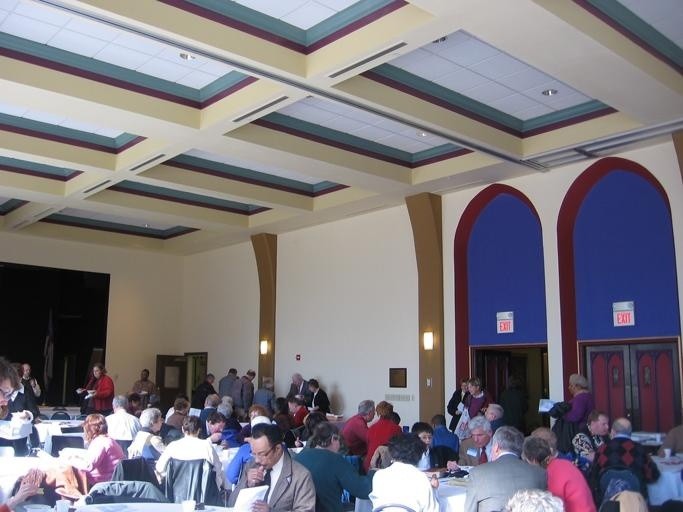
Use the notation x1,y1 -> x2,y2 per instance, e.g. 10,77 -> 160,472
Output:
261,468 -> 272,504
479,447 -> 487,464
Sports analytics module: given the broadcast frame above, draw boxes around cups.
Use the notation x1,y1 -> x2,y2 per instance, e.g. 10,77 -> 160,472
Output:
183,501 -> 196,512
56,499 -> 71,512
664,448 -> 671,458
656,433 -> 662,442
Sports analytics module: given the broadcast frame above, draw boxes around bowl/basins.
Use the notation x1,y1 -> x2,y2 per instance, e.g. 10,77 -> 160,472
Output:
87,389 -> 97,395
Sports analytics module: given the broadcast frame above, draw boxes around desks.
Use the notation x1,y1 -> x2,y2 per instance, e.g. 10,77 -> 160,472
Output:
645,453 -> 683,505
353,467 -> 477,512
1,419 -> 87,454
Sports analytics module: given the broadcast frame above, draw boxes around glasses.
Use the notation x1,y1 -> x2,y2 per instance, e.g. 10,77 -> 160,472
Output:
155,418 -> 164,424
248,442 -> 282,458
0,388 -> 10,401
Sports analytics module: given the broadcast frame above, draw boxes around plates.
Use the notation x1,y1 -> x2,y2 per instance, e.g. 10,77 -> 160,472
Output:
138,392 -> 147,395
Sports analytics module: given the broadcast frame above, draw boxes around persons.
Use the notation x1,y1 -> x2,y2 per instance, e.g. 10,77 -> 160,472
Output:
530,426 -> 575,463
657,423 -> 683,457
1,395 -> 460,512
560,409 -> 610,479
591,417 -> 661,507
559,374 -> 596,432
447,376 -> 504,466
192,374 -> 217,408
219,368 -> 275,413
287,373 -> 330,415
132,368 -> 154,409
464,426 -> 547,512
1,360 -> 42,455
521,433 -> 597,511
78,363 -> 114,415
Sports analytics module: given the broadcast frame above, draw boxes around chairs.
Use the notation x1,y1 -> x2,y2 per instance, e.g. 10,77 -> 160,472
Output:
38,412 -> 71,421
7,471 -> 172,503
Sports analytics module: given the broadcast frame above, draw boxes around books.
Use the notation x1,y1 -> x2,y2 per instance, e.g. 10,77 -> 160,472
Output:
538,399 -> 558,413
